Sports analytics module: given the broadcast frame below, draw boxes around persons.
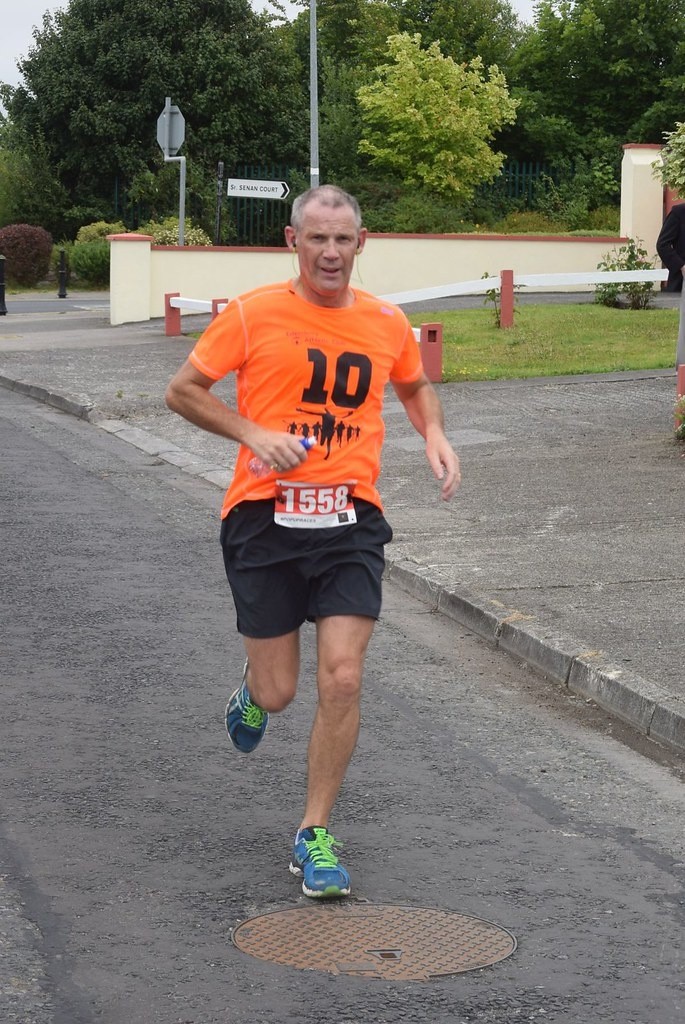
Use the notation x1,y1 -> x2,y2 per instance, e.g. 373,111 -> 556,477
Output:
656,202 -> 685,371
165,184 -> 461,899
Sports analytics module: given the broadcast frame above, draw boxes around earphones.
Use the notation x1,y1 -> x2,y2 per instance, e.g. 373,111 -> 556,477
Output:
356,242 -> 360,250
292,238 -> 296,247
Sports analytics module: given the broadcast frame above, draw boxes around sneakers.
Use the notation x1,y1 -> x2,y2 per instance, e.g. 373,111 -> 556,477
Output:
225,658 -> 270,754
289,824 -> 351,898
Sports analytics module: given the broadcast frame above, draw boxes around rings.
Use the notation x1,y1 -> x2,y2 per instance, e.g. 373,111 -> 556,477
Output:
458,474 -> 461,476
271,463 -> 279,469
